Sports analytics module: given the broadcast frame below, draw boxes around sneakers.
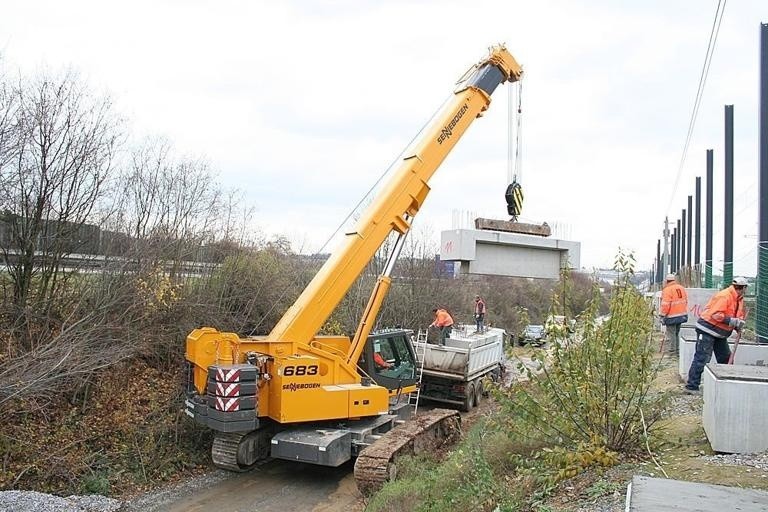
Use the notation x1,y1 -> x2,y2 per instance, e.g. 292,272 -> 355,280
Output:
683,387 -> 701,395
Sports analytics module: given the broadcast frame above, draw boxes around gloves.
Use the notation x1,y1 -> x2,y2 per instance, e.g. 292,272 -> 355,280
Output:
472,314 -> 479,319
428,324 -> 432,328
728,317 -> 745,331
659,317 -> 666,324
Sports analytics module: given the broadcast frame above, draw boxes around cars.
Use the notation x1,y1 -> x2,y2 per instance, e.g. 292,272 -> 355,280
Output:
545,315 -> 570,337
518,325 -> 547,347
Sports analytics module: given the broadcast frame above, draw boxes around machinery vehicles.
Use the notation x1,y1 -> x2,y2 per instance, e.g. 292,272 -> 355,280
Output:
182,42 -> 524,494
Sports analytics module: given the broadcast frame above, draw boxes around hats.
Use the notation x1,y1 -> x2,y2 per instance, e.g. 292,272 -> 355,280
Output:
731,276 -> 751,287
664,274 -> 675,281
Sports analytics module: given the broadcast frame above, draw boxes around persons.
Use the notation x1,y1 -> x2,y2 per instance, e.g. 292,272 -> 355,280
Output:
473,295 -> 486,335
685,276 -> 751,395
658,273 -> 688,360
429,308 -> 454,347
373,351 -> 391,369
